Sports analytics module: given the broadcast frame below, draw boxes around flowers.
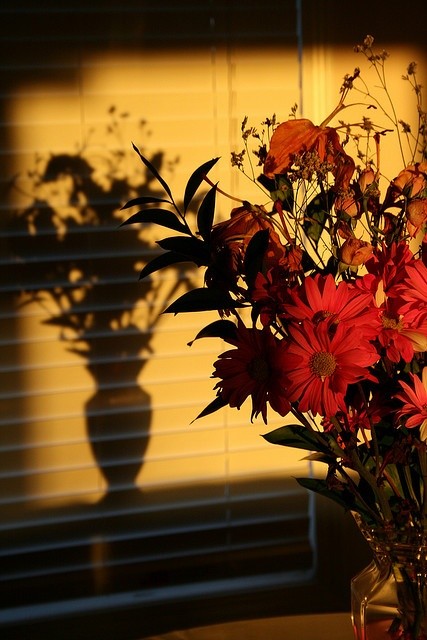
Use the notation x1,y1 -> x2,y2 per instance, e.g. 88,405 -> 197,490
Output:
123,31 -> 424,639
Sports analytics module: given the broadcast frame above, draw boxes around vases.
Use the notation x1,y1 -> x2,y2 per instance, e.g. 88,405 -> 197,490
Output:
347,528 -> 427,640
